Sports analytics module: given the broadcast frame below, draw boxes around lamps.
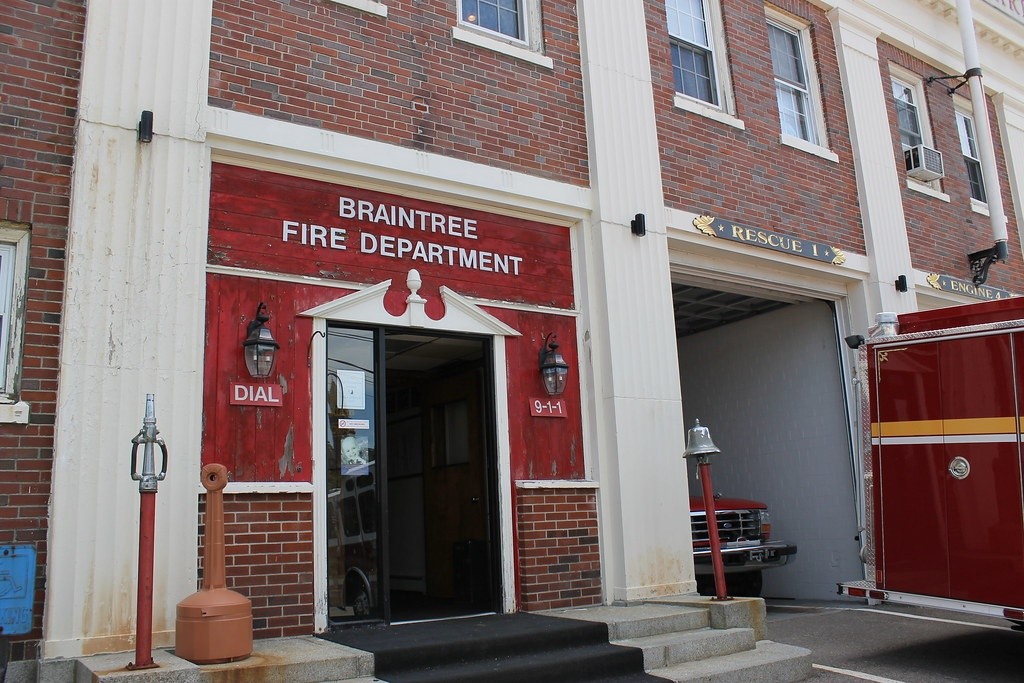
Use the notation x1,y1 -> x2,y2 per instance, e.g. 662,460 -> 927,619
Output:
539,331 -> 569,394
895,275 -> 907,292
138,110 -> 154,142
631,214 -> 646,237
242,300 -> 280,378
844,335 -> 866,349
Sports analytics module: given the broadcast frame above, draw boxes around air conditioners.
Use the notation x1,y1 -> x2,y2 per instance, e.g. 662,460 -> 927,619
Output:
904,144 -> 944,182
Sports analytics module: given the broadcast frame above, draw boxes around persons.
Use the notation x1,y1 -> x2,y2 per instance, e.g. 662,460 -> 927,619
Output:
341,437 -> 369,465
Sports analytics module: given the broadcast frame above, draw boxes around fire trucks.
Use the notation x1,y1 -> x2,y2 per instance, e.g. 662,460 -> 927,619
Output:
688,492 -> 798,599
837,296 -> 1024,633
